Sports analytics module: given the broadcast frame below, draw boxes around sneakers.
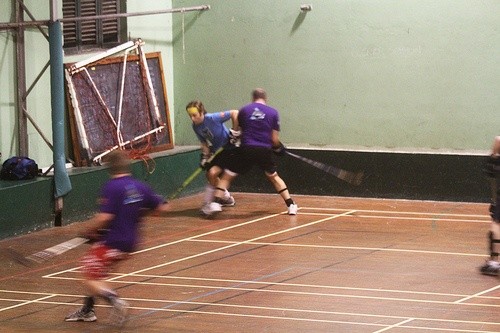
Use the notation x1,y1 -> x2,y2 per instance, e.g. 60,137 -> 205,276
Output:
63,308 -> 96,322
216,197 -> 235,207
200,203 -> 221,217
107,300 -> 129,330
288,204 -> 298,215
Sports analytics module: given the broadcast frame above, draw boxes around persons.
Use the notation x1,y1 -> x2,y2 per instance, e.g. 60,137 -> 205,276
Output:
186,102 -> 240,217
65,151 -> 168,329
202,88 -> 297,221
478,135 -> 500,275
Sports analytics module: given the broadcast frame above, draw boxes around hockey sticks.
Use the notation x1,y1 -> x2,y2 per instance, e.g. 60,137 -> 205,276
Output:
152,147 -> 223,216
286,151 -> 372,188
9,237 -> 93,268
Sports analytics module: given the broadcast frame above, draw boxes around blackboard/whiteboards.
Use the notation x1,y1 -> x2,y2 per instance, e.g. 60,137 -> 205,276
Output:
64,52 -> 174,166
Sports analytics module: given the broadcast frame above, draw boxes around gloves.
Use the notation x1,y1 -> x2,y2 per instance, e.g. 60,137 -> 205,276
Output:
199,151 -> 210,171
228,129 -> 242,148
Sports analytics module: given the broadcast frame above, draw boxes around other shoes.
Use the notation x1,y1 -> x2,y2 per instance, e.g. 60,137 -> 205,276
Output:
2,156 -> 37,181
481,260 -> 500,275
